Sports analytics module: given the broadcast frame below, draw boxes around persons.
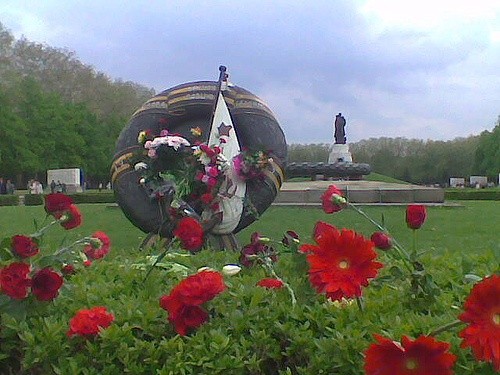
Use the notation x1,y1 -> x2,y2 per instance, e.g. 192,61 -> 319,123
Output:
431,179 -> 495,189
0,175 -> 112,199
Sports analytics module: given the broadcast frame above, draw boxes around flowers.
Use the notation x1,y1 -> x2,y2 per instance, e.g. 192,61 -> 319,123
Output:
1,122 -> 500,375
